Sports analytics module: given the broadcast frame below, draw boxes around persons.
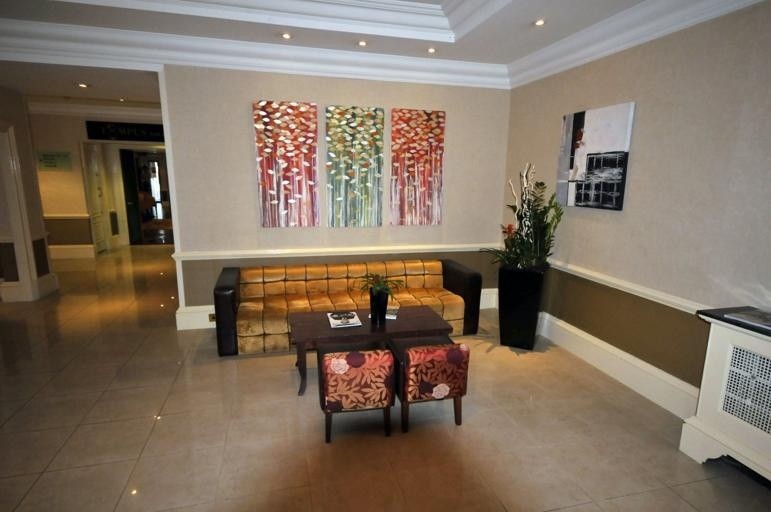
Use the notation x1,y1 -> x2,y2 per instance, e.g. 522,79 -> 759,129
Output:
134,179 -> 155,222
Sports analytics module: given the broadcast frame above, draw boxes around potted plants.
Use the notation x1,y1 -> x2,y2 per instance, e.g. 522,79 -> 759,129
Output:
477,160 -> 565,349
357,272 -> 405,331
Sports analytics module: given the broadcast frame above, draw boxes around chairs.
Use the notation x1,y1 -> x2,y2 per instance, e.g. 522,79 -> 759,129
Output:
316,341 -> 393,444
397,345 -> 469,432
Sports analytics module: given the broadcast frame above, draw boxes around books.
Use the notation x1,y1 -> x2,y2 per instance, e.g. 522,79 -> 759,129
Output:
368,305 -> 400,319
724,311 -> 771,329
327,310 -> 362,329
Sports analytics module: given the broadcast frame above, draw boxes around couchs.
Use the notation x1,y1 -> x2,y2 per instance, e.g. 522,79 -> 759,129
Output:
212,258 -> 484,357
